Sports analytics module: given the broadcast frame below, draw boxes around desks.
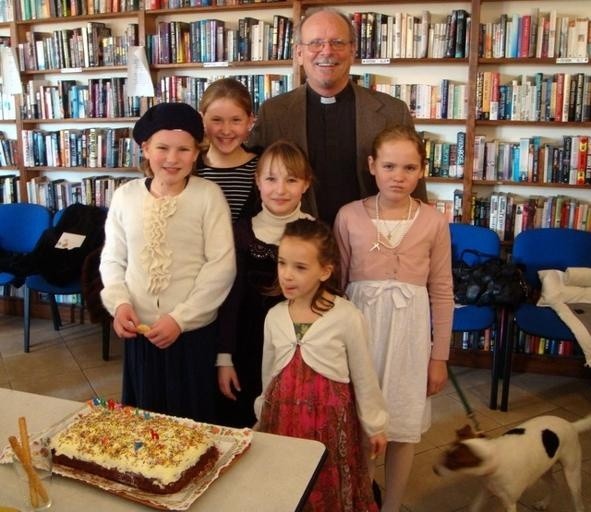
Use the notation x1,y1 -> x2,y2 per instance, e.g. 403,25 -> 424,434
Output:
0,387 -> 328,511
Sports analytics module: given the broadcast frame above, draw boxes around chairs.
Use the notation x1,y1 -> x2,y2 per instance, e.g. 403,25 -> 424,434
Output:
22,205 -> 111,362
500,227 -> 590,412
448,223 -> 503,410
1,203 -> 62,353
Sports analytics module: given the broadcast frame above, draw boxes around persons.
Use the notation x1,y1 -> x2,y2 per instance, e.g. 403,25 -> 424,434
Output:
252,217 -> 391,512
215,138 -> 319,425
330,125 -> 455,512
99,102 -> 238,424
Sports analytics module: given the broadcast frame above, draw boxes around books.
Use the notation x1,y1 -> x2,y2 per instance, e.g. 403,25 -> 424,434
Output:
0,0 -> 590,359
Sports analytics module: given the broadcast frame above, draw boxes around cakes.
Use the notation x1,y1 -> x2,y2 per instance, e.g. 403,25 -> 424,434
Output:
48,404 -> 219,495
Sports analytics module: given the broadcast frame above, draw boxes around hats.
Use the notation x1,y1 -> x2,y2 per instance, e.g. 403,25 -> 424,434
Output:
132,103 -> 204,143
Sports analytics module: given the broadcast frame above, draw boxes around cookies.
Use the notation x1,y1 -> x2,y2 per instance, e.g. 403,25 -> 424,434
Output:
10,415 -> 48,509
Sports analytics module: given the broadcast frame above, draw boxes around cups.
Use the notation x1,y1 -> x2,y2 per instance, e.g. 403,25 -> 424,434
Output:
3,443 -> 53,509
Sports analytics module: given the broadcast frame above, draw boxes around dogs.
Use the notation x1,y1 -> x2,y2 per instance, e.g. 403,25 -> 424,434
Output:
432,414 -> 591,512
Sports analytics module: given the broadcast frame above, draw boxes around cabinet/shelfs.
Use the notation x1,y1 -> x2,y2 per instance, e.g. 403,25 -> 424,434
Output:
0,1 -> 590,379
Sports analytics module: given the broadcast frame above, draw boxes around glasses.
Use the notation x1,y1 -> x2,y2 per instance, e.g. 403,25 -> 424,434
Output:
299,42 -> 353,51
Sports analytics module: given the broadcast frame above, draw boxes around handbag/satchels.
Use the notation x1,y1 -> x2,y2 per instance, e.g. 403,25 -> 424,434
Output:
453,250 -> 542,305
